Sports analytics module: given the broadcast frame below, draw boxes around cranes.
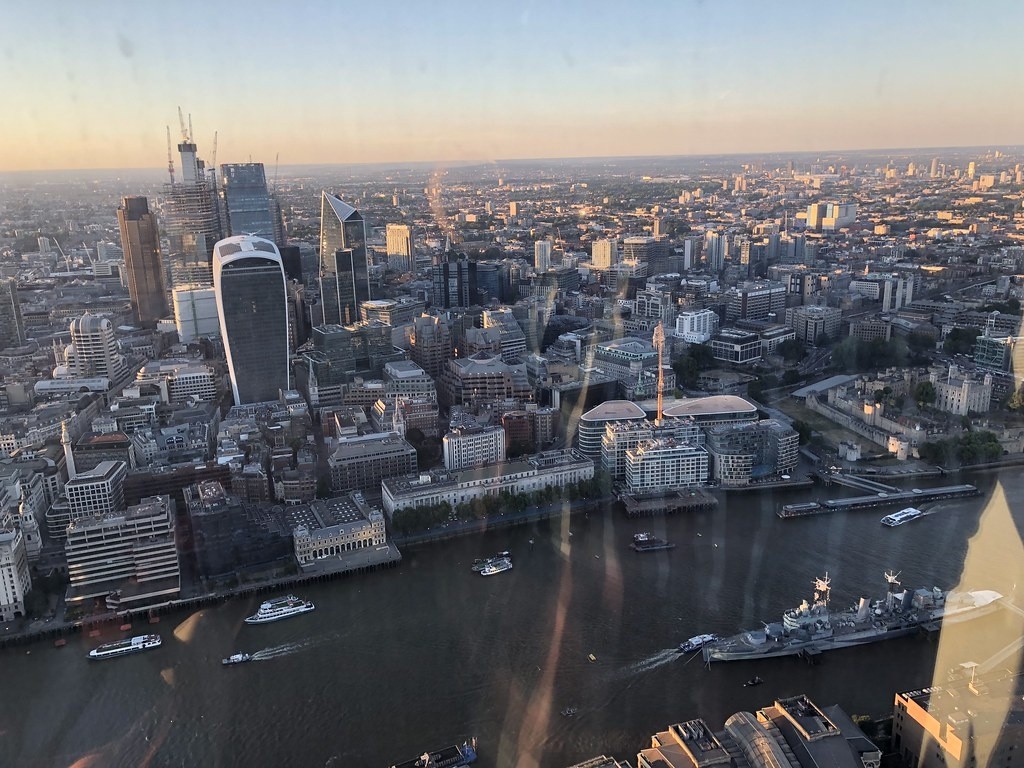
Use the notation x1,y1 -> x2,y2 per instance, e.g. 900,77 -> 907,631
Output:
164,105 -> 220,183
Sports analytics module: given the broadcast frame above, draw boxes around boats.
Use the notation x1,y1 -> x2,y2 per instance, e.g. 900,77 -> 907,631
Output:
389,745 -> 477,768
221,653 -> 250,665
679,633 -> 716,651
473,551 -> 514,576
701,570 -> 1006,664
880,507 -> 922,527
630,531 -> 676,552
244,594 -> 316,625
85,633 -> 161,662
